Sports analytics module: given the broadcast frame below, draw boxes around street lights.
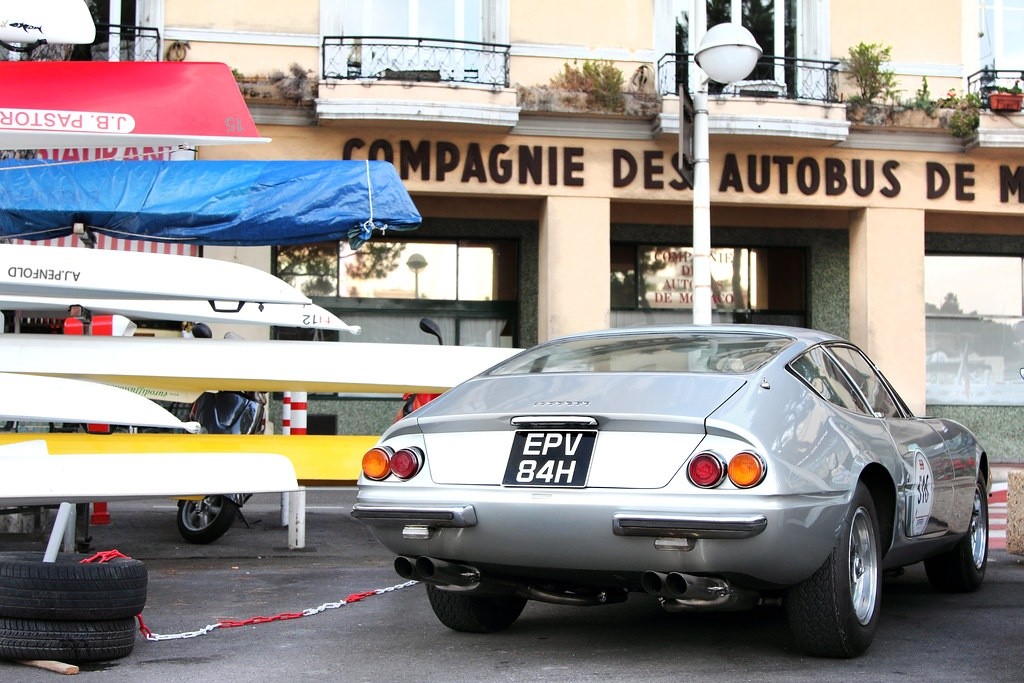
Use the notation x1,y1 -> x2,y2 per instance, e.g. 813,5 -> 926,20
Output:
694,22 -> 763,327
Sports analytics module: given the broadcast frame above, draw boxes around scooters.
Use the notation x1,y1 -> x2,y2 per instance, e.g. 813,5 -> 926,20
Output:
178,321 -> 267,545
395,319 -> 444,422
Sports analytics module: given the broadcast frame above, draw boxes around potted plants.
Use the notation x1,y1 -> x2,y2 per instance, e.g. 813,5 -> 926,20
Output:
510,58 -> 661,121
988,81 -> 1024,111
842,42 -> 970,135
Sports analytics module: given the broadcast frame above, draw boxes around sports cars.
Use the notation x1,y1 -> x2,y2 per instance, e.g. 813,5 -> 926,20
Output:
349,317 -> 993,657
